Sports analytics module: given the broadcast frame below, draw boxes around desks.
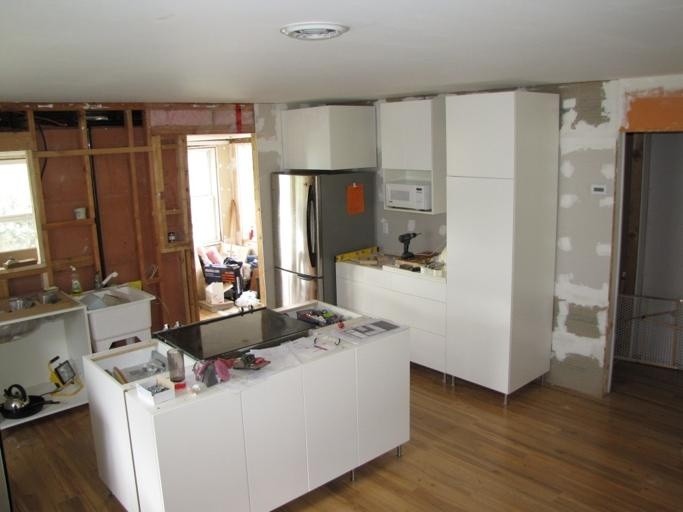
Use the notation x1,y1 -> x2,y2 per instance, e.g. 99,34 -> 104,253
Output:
250,268 -> 259,291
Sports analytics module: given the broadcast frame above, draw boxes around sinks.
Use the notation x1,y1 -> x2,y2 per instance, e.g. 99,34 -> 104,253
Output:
75,285 -> 157,341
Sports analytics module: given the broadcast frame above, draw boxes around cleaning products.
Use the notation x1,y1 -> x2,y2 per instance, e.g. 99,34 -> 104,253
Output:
69,265 -> 83,295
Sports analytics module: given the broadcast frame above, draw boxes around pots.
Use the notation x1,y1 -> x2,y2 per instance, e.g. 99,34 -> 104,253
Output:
0,395 -> 60,419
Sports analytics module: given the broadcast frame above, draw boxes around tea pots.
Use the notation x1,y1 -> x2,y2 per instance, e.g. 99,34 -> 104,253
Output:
3,384 -> 30,410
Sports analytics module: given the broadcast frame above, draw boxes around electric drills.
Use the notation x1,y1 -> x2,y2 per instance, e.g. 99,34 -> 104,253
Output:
398,233 -> 422,259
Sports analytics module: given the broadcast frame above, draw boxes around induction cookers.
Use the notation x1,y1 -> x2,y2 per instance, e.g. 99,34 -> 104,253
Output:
152,303 -> 315,364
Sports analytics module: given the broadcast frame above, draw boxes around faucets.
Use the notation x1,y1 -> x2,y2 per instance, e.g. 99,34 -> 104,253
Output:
101,272 -> 119,286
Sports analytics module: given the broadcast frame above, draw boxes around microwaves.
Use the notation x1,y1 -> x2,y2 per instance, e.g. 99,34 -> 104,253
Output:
385,179 -> 432,211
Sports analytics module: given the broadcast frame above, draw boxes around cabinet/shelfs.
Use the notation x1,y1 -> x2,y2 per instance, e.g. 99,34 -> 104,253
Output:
448,92 -> 559,403
83,329 -> 410,512
381,101 -> 445,215
336,261 -> 448,384
283,107 -> 376,169
0,306 -> 92,431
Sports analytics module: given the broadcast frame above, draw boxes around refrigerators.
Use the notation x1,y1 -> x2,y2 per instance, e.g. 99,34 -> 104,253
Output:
270,171 -> 377,310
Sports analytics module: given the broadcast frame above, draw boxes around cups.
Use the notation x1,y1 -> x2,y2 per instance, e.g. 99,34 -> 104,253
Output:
166,350 -> 185,382
74,207 -> 86,219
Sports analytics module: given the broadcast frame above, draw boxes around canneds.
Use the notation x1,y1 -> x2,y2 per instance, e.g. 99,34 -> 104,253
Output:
169,232 -> 176,242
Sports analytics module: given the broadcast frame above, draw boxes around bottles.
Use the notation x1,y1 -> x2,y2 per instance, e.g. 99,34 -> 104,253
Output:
169,232 -> 176,243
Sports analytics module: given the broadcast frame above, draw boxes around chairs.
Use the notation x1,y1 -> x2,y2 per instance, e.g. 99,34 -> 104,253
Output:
198,247 -> 240,298
